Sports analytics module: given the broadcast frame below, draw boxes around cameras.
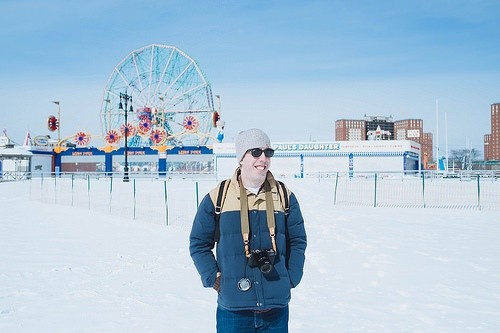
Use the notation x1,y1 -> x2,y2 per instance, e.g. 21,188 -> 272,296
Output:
248,248 -> 275,278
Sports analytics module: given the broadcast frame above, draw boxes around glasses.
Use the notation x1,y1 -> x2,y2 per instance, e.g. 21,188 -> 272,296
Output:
241,147 -> 275,161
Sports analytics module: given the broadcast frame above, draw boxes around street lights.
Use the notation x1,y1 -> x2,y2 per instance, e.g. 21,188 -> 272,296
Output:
119,88 -> 133,183
158,96 -> 164,132
215,94 -> 220,135
52,101 -> 60,146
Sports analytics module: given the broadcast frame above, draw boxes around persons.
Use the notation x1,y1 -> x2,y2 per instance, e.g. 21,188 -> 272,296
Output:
189,129 -> 307,333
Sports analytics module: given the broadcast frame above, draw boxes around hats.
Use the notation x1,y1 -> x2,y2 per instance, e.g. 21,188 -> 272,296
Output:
235,128 -> 271,165
